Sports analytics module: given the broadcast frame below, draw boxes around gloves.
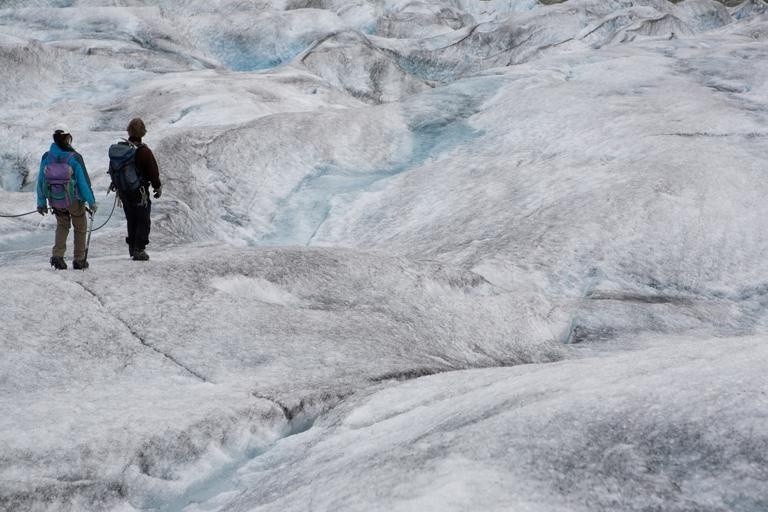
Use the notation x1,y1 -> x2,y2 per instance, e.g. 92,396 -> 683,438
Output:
37,206 -> 48,216
91,204 -> 98,211
153,187 -> 162,199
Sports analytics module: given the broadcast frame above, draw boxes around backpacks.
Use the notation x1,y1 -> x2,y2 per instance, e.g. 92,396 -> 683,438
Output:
108,142 -> 140,193
43,152 -> 77,209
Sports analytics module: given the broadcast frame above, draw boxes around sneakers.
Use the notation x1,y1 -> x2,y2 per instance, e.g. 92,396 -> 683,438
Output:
73,260 -> 89,269
129,246 -> 135,256
50,255 -> 67,269
134,248 -> 149,260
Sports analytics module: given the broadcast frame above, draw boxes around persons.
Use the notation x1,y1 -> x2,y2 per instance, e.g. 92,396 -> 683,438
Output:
108,117 -> 163,262
36,123 -> 97,271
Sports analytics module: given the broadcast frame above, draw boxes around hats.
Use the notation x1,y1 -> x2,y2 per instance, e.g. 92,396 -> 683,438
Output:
126,118 -> 146,137
52,124 -> 71,135
